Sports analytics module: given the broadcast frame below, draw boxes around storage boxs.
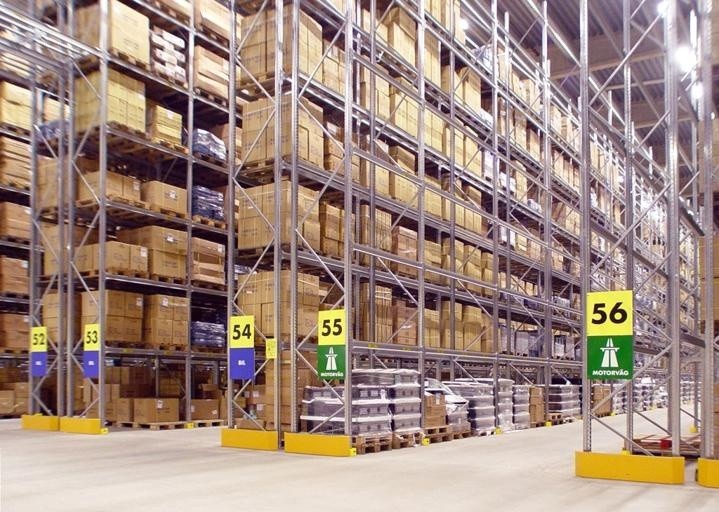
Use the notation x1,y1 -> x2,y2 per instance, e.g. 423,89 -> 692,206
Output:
233,0 -> 718,458
2,0 -> 238,438
